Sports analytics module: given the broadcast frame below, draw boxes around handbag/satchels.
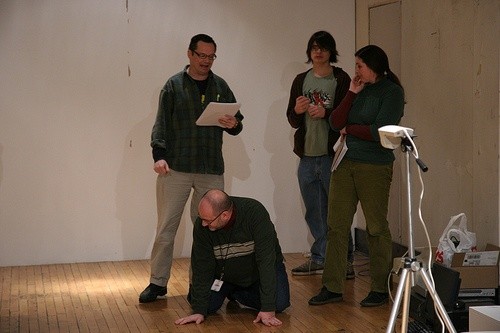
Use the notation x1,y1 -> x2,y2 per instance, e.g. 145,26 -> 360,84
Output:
434,213 -> 478,268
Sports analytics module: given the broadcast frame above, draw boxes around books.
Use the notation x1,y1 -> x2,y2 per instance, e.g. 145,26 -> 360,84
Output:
196,103 -> 241,128
331,134 -> 349,172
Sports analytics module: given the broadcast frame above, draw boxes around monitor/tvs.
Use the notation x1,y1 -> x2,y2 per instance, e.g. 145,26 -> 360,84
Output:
424,263 -> 461,325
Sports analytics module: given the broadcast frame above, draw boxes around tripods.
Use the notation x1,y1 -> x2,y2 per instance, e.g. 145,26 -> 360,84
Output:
386,133 -> 459,333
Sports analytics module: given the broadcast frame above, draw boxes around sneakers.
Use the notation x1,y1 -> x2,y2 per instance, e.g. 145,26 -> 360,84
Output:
360,291 -> 389,305
345,263 -> 355,279
308,286 -> 343,304
187,285 -> 191,304
291,261 -> 324,274
139,283 -> 167,302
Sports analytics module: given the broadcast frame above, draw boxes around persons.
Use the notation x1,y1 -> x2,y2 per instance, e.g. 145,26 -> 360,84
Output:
139,34 -> 243,303
308,46 -> 405,307
287,32 -> 356,280
175,188 -> 291,327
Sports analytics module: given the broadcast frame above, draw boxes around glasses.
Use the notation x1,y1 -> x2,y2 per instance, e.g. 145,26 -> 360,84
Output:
196,209 -> 228,224
193,49 -> 216,60
312,45 -> 320,51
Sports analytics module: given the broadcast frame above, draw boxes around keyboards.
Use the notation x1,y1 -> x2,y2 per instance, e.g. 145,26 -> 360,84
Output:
408,319 -> 434,333
